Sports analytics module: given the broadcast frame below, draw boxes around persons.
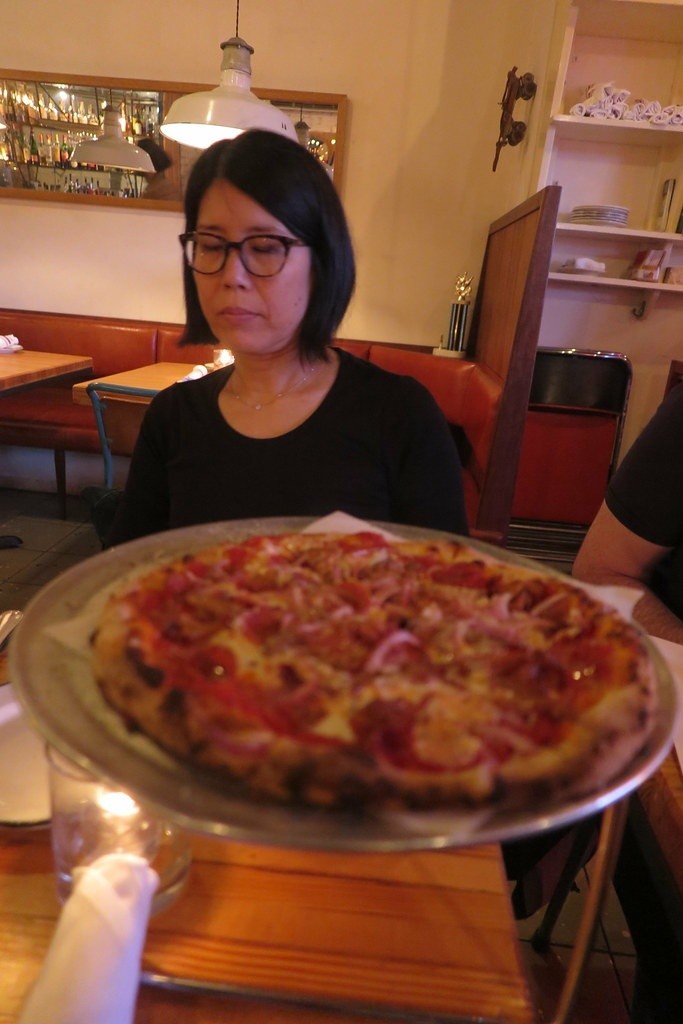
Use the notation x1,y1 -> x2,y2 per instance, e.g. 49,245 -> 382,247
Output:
117,126 -> 470,535
572,382 -> 683,1023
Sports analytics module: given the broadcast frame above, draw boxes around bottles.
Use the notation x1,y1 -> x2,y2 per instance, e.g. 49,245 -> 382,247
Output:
0,79 -> 154,198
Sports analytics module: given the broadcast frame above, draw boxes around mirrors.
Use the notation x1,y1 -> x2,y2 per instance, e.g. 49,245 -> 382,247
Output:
0,69 -> 352,227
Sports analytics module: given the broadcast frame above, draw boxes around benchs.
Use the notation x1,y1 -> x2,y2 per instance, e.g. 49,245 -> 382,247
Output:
0,307 -> 506,543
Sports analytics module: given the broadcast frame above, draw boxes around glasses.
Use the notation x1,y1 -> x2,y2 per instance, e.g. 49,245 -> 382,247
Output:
179,232 -> 311,277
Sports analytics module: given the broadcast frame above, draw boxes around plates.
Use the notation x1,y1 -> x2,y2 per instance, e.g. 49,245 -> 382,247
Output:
570,205 -> 630,229
560,267 -> 606,276
8,515 -> 679,851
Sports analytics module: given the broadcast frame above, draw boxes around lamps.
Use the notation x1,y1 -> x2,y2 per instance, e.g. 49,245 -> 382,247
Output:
64,98 -> 158,175
158,0 -> 299,150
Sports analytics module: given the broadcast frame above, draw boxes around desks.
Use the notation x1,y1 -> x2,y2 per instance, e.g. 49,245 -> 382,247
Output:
0,349 -> 91,391
1,650 -> 537,1024
72,361 -> 204,496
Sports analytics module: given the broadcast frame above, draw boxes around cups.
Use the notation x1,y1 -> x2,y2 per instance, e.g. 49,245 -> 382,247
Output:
44,741 -> 194,920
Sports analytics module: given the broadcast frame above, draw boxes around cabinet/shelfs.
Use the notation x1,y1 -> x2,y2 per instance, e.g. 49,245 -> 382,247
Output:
522,0 -> 683,314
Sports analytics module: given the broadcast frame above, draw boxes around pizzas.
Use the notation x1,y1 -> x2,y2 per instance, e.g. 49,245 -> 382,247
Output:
87,530 -> 659,812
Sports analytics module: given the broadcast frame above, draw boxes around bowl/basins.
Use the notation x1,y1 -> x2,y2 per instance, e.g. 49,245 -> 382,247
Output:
0,344 -> 23,353
0,682 -> 52,832
